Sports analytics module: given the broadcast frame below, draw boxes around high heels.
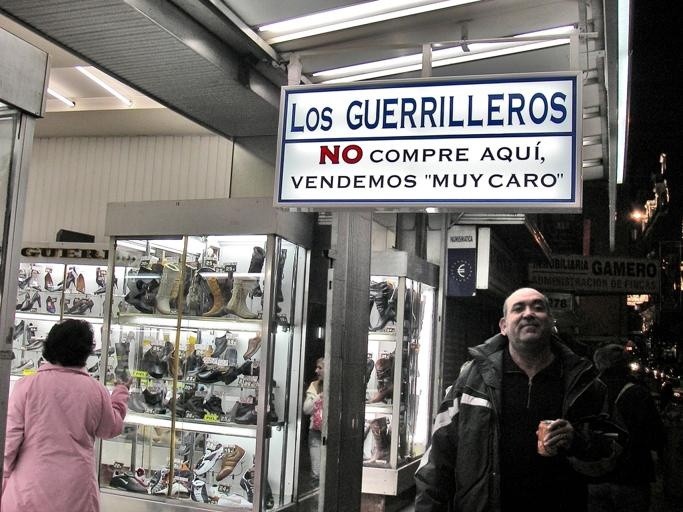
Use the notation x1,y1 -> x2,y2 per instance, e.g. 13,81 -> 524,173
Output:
18,264 -> 118,316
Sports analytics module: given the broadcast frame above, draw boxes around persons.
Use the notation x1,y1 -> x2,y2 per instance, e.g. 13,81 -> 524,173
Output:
303,356 -> 326,480
407,283 -> 631,512
584,343 -> 670,512
0,316 -> 136,512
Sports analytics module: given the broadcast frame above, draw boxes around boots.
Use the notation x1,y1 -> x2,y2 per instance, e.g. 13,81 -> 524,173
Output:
123,246 -> 284,319
363,279 -> 426,467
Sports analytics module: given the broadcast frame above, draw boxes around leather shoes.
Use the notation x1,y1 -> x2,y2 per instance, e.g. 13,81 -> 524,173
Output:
11,320 -> 115,378
126,331 -> 279,424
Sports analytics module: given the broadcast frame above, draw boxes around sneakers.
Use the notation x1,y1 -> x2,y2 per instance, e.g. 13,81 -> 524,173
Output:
109,424 -> 273,511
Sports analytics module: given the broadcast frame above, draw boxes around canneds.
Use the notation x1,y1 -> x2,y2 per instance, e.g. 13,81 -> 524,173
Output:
537,419 -> 558,457
